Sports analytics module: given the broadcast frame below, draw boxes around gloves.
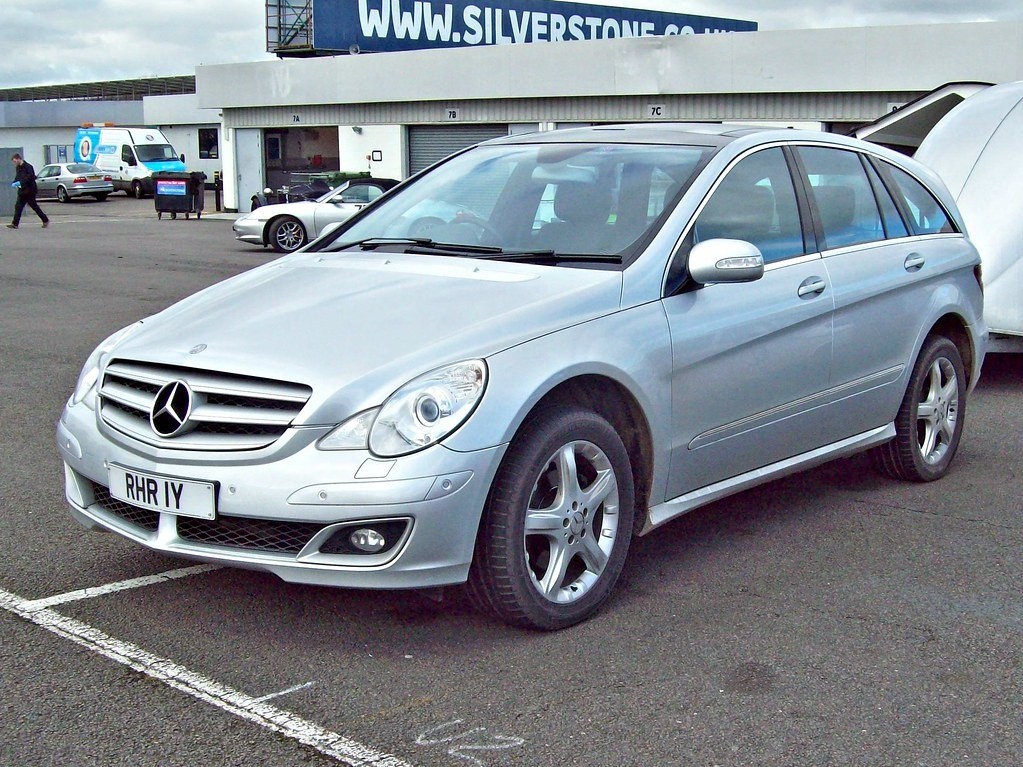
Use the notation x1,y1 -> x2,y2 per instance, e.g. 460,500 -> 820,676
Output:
12,181 -> 20,188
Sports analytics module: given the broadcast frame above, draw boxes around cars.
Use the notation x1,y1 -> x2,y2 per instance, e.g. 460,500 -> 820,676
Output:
32,162 -> 115,203
231,178 -> 404,253
54,124 -> 992,633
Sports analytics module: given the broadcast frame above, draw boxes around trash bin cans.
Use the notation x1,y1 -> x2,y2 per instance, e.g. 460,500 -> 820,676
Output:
151,170 -> 209,220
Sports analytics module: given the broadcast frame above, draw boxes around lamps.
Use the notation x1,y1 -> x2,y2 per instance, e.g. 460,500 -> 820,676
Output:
352,126 -> 362,134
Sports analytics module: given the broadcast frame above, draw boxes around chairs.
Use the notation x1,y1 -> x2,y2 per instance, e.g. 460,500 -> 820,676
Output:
665,178 -> 856,244
533,178 -> 625,253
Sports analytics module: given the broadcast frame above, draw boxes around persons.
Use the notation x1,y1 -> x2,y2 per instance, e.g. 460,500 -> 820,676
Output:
7,153 -> 50,229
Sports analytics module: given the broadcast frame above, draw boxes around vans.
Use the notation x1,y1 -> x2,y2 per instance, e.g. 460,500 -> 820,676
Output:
73,122 -> 186,199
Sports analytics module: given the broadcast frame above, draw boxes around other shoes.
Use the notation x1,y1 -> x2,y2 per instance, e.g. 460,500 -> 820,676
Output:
6,225 -> 19,229
41,220 -> 51,229
